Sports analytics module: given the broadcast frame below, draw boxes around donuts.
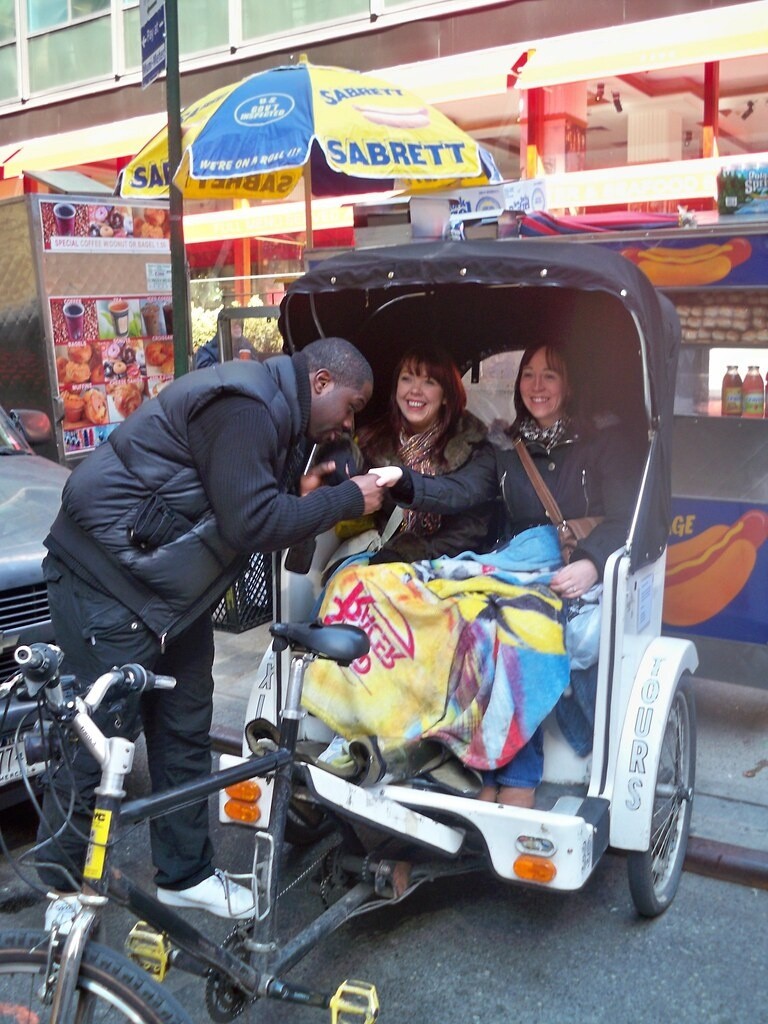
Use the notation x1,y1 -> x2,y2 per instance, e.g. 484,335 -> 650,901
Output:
88,205 -> 133,238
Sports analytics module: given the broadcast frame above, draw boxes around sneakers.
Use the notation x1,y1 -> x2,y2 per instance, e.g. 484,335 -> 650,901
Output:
44,895 -> 83,936
157,868 -> 257,919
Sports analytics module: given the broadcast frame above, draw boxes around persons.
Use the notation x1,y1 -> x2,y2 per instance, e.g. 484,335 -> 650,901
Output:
366,330 -> 640,806
307,338 -> 495,777
33,331 -> 388,939
193,310 -> 261,372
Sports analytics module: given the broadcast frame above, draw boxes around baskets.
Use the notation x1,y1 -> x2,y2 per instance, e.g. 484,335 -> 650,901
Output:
211,549 -> 272,633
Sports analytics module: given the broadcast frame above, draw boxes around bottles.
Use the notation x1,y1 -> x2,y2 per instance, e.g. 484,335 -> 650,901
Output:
765,371 -> 768,418
720,366 -> 743,415
65,427 -> 105,448
742,366 -> 764,419
717,162 -> 768,216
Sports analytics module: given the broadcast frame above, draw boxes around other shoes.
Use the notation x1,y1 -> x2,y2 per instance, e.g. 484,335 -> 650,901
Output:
475,784 -> 534,809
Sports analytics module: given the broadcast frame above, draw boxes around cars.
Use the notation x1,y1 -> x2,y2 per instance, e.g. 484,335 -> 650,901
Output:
1,406 -> 84,812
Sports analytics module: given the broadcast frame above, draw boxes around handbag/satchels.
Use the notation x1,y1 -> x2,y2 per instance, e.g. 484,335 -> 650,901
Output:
557,515 -> 608,567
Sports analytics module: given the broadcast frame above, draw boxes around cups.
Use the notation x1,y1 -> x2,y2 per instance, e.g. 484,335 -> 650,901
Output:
63,303 -> 86,341
142,305 -> 161,337
54,203 -> 76,236
109,300 -> 130,337
163,304 -> 174,335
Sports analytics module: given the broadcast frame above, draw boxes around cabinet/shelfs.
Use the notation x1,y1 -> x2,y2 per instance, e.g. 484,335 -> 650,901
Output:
660,284 -> 768,646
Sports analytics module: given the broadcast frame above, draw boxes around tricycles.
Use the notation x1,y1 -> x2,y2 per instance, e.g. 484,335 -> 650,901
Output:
0,239 -> 701,1024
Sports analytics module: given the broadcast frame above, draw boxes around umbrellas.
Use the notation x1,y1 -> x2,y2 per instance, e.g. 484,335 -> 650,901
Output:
113,49 -> 505,198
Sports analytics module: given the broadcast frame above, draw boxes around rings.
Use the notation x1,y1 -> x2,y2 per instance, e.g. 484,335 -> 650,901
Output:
572,586 -> 576,591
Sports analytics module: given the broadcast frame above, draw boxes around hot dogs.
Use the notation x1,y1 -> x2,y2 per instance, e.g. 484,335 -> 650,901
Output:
354,103 -> 429,129
662,510 -> 767,628
620,238 -> 752,287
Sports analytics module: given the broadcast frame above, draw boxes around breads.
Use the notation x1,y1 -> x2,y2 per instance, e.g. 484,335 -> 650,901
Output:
57,341 -> 174,425
134,208 -> 171,239
